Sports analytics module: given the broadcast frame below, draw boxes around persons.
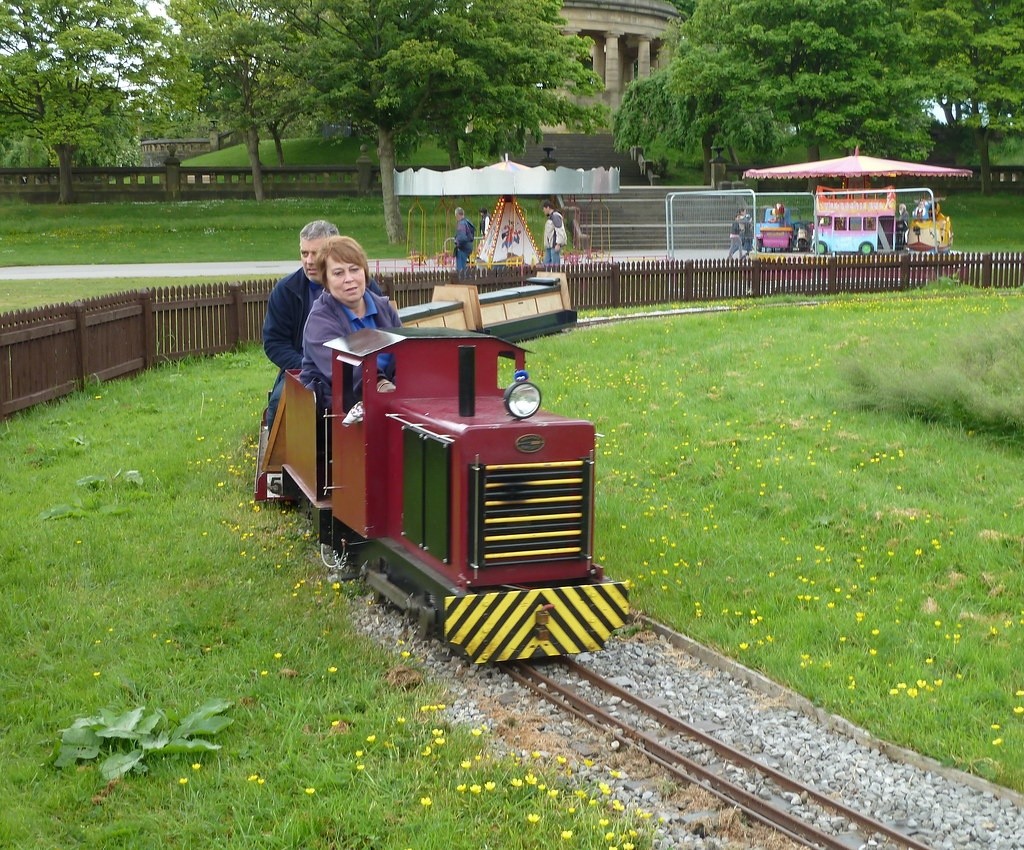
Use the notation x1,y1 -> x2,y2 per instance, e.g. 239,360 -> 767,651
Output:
728,208 -> 752,263
262,219 -> 383,442
899,204 -> 908,221
454,207 -> 476,271
299,236 -> 404,412
479,208 -> 491,236
541,199 -> 567,265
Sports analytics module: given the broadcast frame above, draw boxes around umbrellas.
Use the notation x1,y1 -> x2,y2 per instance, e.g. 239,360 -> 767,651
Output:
746,145 -> 973,179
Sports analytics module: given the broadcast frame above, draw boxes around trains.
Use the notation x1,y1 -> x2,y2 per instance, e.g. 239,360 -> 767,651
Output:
259,272 -> 638,668
